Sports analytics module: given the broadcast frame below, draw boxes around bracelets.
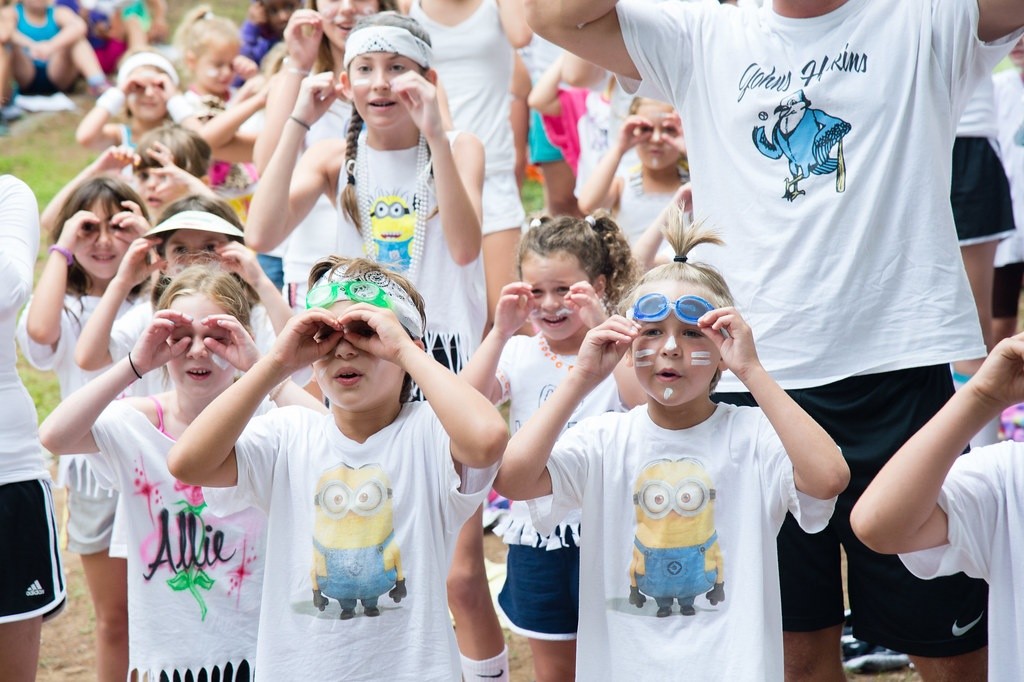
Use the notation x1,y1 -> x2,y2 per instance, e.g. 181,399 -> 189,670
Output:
48,244 -> 74,265
286,67 -> 309,76
289,114 -> 310,130
128,352 -> 142,379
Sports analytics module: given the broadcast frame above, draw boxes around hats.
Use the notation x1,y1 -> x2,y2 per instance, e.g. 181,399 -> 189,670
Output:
142,211 -> 244,236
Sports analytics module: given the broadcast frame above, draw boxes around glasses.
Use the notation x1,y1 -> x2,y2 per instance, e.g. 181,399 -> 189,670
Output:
625,292 -> 729,339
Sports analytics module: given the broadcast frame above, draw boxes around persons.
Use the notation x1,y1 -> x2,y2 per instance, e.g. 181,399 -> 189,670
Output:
0,0 -> 1024,682
